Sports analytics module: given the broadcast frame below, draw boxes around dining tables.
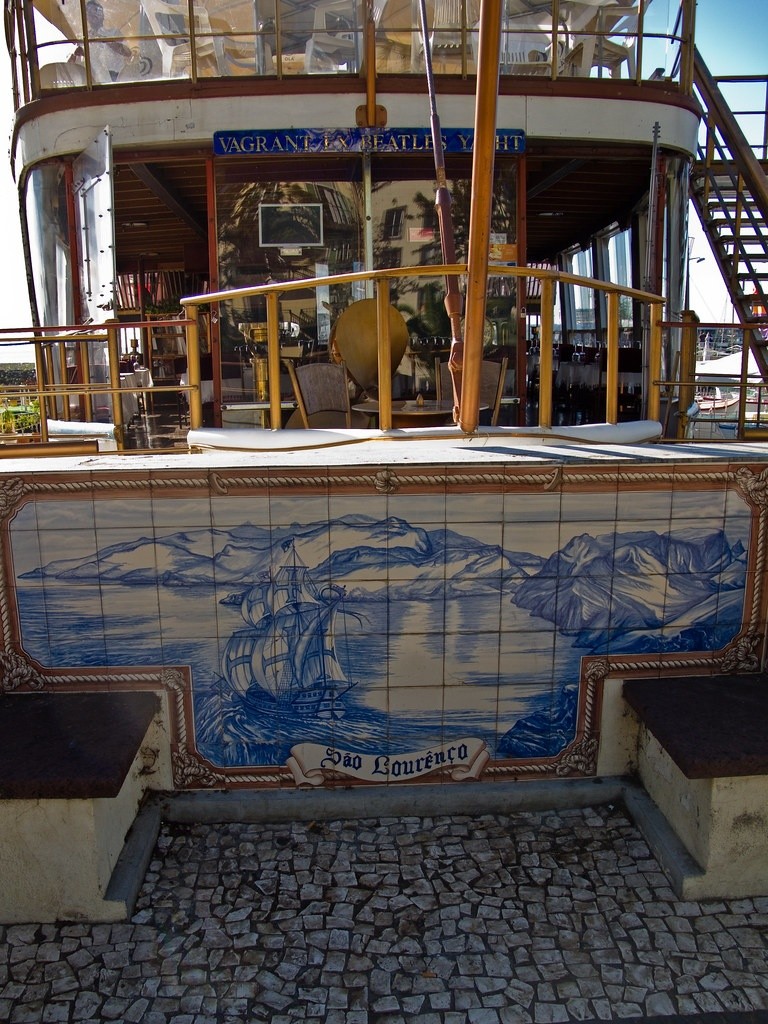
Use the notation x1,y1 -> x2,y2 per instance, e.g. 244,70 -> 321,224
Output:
120,366 -> 244,431
351,398 -> 489,426
527,352 -> 643,410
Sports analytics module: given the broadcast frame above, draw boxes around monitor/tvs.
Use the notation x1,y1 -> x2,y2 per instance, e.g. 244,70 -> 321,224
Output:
258,203 -> 323,247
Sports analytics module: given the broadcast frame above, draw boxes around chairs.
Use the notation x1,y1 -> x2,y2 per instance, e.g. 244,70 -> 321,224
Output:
286,357 -> 353,428
434,356 -> 509,425
